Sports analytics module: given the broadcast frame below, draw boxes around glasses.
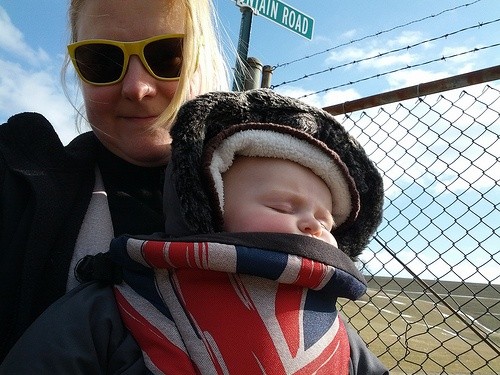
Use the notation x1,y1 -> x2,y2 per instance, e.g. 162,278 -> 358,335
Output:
67,35 -> 184,84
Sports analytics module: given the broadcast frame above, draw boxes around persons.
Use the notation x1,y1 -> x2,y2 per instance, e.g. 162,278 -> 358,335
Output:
0,87 -> 392,375
0,0 -> 261,375
